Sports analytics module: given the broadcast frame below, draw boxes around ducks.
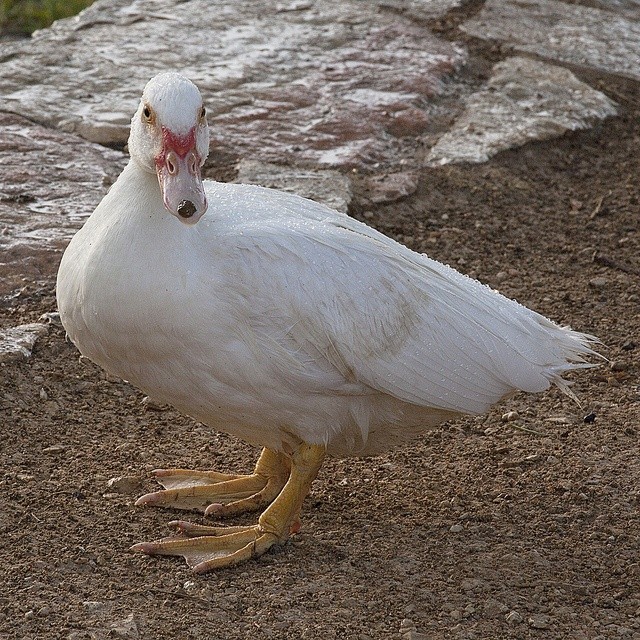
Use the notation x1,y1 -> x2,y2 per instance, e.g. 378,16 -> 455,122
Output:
56,72 -> 610,578
